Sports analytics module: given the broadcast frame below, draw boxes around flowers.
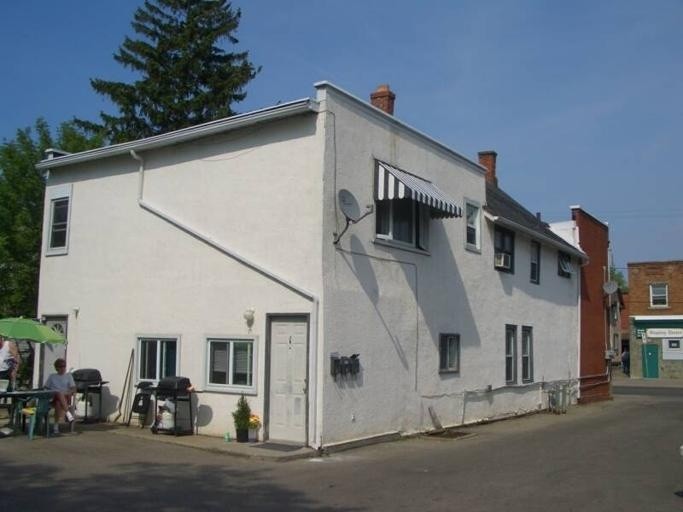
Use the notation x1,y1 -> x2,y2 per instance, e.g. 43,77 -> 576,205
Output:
249,415 -> 260,429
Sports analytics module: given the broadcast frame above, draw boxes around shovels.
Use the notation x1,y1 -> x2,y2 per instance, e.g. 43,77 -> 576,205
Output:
113,348 -> 134,423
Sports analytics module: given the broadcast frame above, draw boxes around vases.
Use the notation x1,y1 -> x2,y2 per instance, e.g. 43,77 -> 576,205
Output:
248,428 -> 256,440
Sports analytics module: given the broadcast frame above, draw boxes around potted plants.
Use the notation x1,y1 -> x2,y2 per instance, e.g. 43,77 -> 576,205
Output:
231,391 -> 251,442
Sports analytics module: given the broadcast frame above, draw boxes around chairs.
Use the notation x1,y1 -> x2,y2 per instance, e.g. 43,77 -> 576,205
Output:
0,369 -> 109,440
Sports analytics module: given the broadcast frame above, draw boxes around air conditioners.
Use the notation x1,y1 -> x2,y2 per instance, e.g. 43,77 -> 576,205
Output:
495,252 -> 511,270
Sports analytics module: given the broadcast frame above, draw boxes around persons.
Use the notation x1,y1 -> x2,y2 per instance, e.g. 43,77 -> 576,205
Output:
620,349 -> 630,374
43,359 -> 76,436
0,335 -> 21,427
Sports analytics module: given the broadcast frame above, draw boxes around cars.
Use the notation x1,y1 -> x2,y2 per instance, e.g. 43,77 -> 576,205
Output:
604,351 -> 621,366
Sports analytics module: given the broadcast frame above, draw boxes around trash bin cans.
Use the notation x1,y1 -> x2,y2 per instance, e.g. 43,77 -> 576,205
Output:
71,369 -> 110,424
151,377 -> 194,436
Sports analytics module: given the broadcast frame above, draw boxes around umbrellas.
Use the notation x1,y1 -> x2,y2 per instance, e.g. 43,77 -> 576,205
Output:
0,314 -> 67,343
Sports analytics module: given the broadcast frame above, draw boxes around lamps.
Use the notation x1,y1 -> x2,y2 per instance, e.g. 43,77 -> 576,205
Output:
244,307 -> 254,320
